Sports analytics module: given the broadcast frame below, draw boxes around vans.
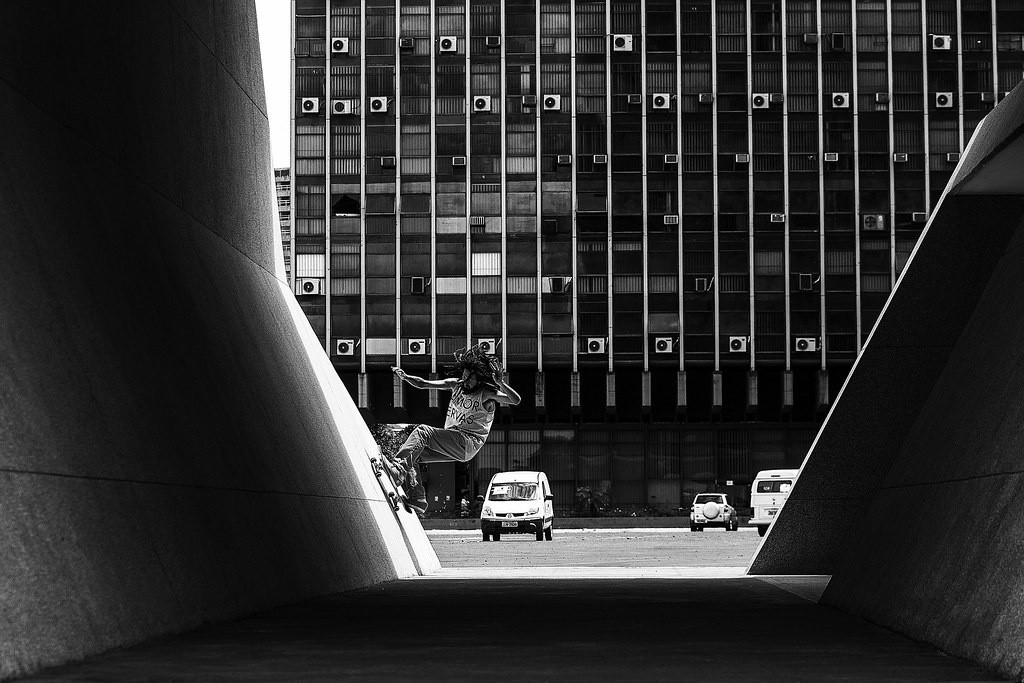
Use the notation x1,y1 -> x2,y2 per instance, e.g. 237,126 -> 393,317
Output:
476,470 -> 555,542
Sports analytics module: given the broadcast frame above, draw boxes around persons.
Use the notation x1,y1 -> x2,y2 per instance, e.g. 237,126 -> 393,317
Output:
380,343 -> 520,513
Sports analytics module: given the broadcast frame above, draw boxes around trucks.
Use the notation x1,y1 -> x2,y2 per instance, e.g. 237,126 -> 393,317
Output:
749,469 -> 799,537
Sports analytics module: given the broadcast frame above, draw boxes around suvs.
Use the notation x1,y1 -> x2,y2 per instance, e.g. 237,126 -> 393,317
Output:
689,493 -> 739,533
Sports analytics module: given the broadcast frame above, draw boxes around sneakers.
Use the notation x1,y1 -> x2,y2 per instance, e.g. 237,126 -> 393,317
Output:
381,459 -> 404,489
402,495 -> 428,513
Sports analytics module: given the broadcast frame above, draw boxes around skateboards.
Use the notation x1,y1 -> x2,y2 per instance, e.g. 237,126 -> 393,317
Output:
370,445 -> 413,515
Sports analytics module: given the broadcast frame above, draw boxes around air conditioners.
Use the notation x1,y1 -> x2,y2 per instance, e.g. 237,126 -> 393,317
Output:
440,36 -> 456,51
301,278 -> 319,294
478,339 -> 495,354
796,338 -> 816,351
409,339 -> 425,354
474,96 -> 491,111
337,340 -> 354,355
588,338 -> 604,353
331,37 -> 349,52
302,97 -> 319,113
655,337 -> 672,353
752,93 -> 769,108
653,93 -> 669,108
730,336 -> 746,352
544,94 -> 560,110
614,34 -> 633,51
936,92 -> 952,106
370,97 -> 388,112
832,92 -> 849,108
333,100 -> 351,114
933,35 -> 950,49
864,214 -> 884,230
